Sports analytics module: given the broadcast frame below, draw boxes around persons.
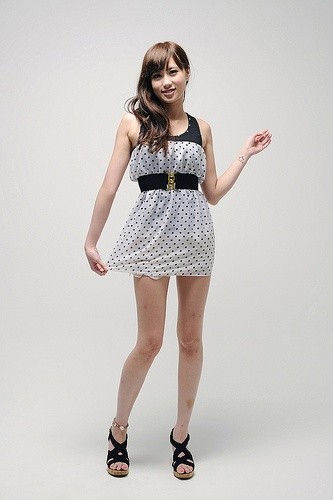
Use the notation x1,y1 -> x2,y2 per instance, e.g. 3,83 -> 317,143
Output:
84,41 -> 272,479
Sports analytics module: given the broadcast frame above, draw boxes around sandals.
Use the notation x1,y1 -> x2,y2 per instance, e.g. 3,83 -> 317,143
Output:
106,427 -> 129,477
170,428 -> 196,479
